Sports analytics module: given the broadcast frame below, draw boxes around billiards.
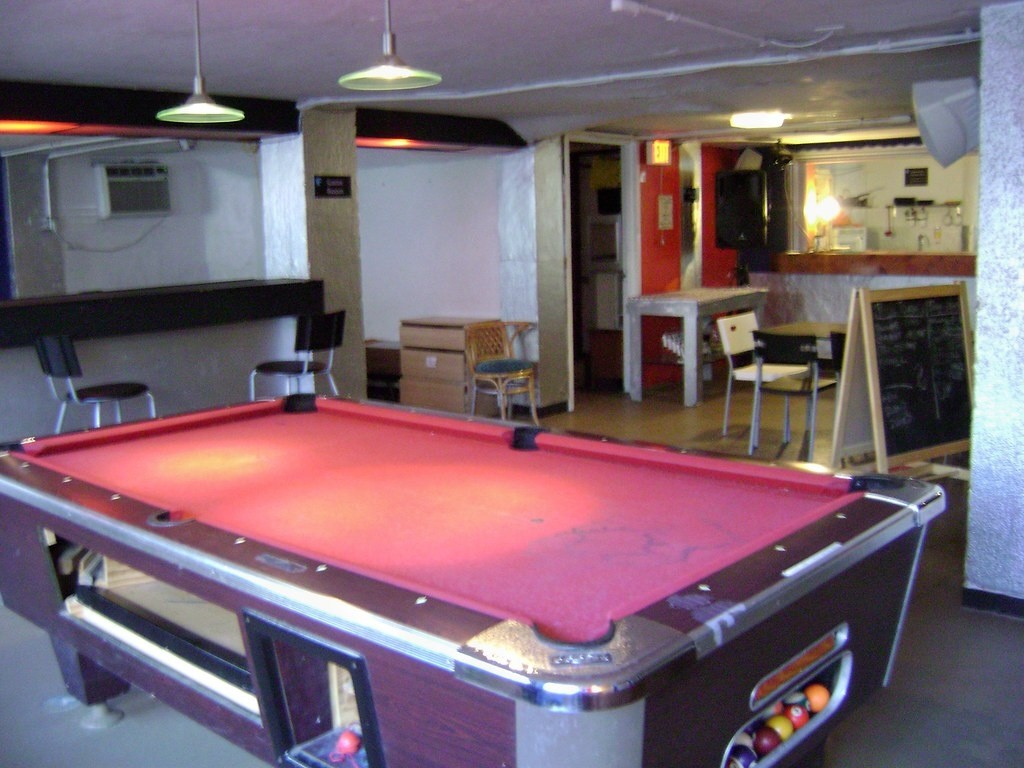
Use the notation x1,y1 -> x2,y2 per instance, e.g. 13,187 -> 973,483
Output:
723,685 -> 830,768
339,731 -> 361,755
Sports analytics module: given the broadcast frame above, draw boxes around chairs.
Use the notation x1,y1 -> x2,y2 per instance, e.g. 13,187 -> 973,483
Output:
718,307 -> 809,447
749,326 -> 824,451
831,327 -> 871,469
31,329 -> 157,435
245,307 -> 346,400
460,315 -> 540,425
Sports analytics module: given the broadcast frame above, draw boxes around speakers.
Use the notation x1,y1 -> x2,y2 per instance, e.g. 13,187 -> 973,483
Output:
716,168 -> 788,254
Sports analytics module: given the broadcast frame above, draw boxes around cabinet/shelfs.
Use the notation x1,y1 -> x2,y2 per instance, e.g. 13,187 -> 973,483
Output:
397,312 -> 503,415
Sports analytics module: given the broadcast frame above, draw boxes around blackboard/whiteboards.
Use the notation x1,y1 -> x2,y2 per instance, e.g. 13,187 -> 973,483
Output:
860,281 -> 973,469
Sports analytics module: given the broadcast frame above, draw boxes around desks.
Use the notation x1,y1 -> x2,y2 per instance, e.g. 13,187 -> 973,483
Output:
0,276 -> 324,346
754,313 -> 850,340
0,387 -> 948,768
625,283 -> 768,406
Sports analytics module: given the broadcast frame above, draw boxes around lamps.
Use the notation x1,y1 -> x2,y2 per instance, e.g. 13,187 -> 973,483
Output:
154,0 -> 244,127
336,0 -> 443,92
